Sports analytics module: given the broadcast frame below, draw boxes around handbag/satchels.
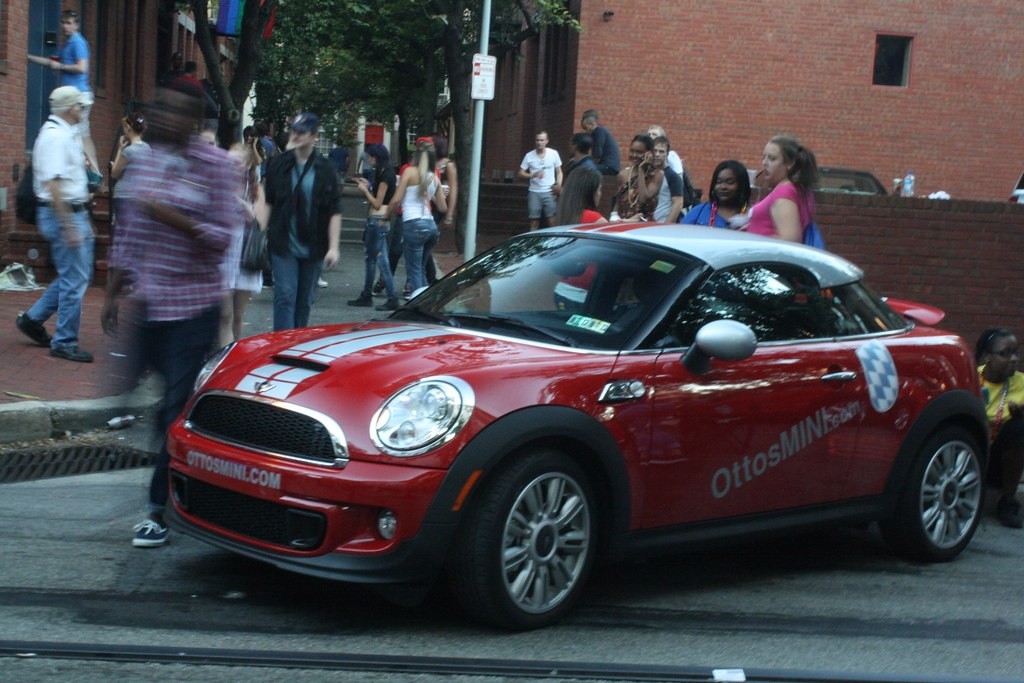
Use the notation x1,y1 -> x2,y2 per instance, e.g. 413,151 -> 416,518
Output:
15,163 -> 34,226
239,213 -> 270,271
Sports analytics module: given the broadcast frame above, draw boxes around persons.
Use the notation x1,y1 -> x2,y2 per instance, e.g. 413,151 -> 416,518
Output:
746,134 -> 821,242
97,50 -> 342,546
49,10 -> 103,180
609,124 -> 684,223
346,133 -> 458,310
554,109 -> 620,310
975,327 -> 1024,527
518,130 -> 563,232
680,159 -> 752,230
16,86 -> 96,360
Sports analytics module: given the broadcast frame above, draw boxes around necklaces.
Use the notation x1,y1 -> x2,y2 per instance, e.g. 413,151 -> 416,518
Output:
709,201 -> 747,227
773,179 -> 791,192
628,165 -> 651,209
978,369 -> 1009,440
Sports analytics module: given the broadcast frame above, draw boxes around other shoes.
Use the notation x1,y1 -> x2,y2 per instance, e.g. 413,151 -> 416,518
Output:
317,278 -> 328,288
402,289 -> 410,299
347,296 -> 372,307
372,290 -> 380,296
375,303 -> 390,310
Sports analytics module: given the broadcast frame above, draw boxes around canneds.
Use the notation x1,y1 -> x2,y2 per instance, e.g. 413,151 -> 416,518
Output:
892,177 -> 903,195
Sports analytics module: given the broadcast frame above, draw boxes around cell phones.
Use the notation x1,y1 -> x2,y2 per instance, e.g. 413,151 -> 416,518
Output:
354,178 -> 360,184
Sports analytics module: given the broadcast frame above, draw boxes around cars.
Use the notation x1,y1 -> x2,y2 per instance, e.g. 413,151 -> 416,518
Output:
817,166 -> 889,197
162,222 -> 993,631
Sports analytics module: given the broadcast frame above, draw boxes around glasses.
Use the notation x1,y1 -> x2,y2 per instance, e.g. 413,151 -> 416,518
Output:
62,10 -> 78,22
75,102 -> 84,109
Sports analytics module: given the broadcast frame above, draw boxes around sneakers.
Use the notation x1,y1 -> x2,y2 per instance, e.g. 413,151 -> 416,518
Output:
131,514 -> 172,548
16,310 -> 53,347
48,339 -> 94,361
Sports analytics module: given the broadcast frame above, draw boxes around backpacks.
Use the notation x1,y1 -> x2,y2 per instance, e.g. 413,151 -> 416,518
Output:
800,188 -> 824,251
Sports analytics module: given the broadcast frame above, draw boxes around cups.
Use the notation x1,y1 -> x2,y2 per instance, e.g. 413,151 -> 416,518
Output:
442,185 -> 449,198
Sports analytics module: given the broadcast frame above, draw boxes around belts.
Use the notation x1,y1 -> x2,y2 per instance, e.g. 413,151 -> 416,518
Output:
38,201 -> 89,211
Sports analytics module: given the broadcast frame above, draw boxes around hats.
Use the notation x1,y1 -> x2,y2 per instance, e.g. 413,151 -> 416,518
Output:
50,86 -> 95,116
416,136 -> 433,149
290,112 -> 319,132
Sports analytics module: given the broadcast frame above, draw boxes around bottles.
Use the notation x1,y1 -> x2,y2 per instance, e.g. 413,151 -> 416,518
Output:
610,211 -> 620,222
892,178 -> 902,197
903,170 -> 915,197
